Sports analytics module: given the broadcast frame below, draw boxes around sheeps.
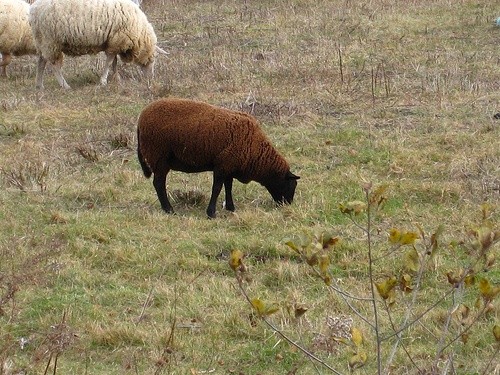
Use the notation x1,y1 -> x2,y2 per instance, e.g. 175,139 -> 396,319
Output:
0,0 -> 171,91
136,97 -> 301,218
